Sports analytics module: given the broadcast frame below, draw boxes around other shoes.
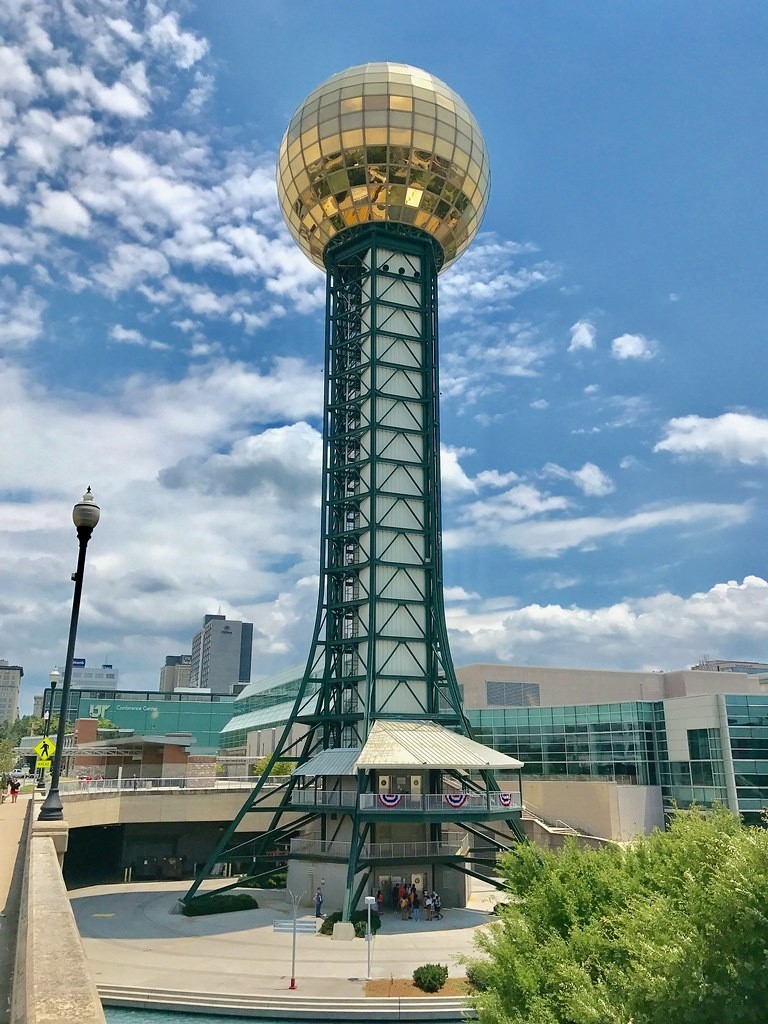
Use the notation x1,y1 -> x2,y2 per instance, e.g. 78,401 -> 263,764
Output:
316,915 -> 320,917
426,919 -> 429,921
430,919 -> 432,921
319,913 -> 323,915
441,916 -> 444,919
11,802 -> 14,803
437,918 -> 439,920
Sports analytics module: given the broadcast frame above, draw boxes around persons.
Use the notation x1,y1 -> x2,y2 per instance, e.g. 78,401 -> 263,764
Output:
392,883 -> 444,922
10,779 -> 21,803
98,774 -> 104,790
313,887 -> 324,919
377,890 -> 384,915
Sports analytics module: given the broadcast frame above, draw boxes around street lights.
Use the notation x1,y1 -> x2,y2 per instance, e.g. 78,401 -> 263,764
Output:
35,485 -> 101,821
36,710 -> 49,781
365,896 -> 376,980
38,666 -> 60,789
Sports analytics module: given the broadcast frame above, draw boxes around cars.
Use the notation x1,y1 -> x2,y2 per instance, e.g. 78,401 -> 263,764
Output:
9,769 -> 34,778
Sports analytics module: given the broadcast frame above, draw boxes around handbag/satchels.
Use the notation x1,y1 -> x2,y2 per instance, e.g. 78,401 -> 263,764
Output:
15,788 -> 20,792
